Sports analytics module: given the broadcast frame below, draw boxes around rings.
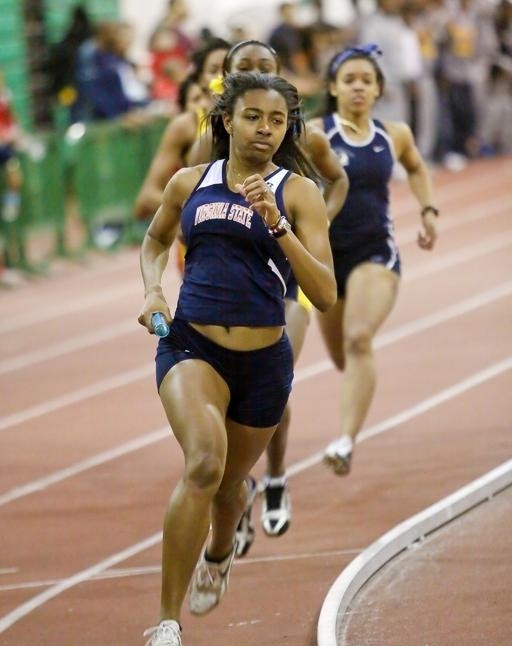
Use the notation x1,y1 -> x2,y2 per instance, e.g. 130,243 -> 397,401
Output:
265,188 -> 268,192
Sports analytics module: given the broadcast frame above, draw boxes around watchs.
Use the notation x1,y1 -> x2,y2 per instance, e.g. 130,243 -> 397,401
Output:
272,221 -> 291,237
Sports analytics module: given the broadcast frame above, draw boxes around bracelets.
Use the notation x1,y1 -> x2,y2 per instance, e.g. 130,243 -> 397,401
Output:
422,206 -> 438,217
270,216 -> 286,230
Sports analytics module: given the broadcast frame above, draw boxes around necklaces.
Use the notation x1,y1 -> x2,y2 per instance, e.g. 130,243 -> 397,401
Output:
233,168 -> 244,181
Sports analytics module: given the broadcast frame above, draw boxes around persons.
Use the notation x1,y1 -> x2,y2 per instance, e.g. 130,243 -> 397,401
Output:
187,37 -> 352,558
134,68 -> 338,644
300,39 -> 442,477
1,0 -> 212,287
269,2 -> 510,180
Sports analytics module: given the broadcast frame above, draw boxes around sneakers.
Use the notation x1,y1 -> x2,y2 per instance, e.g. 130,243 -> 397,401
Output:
261,473 -> 290,536
324,436 -> 353,475
188,533 -> 235,616
235,474 -> 256,558
142,620 -> 181,646
